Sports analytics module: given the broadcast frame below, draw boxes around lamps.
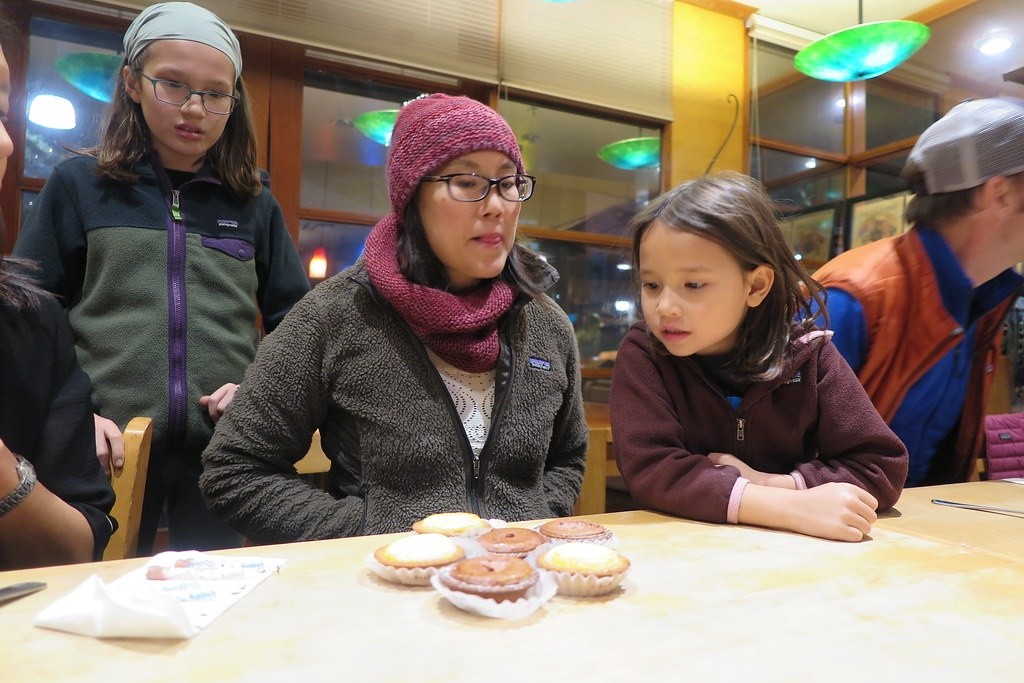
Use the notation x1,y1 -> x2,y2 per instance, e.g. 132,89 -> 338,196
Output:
53,50 -> 123,103
352,109 -> 400,147
309,161 -> 328,278
793,0 -> 931,84
596,127 -> 660,172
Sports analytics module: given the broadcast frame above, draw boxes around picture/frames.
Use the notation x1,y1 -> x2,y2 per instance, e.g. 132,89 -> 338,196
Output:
843,184 -> 917,252
774,199 -> 843,277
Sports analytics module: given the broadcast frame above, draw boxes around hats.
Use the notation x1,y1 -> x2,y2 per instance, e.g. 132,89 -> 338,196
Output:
906,97 -> 1024,194
386,93 -> 525,224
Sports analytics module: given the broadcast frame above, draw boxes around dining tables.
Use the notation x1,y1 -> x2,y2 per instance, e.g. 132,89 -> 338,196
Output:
0,477 -> 1024,683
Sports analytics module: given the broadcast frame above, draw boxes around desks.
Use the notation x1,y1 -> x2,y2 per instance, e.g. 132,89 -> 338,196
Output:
572,401 -> 621,513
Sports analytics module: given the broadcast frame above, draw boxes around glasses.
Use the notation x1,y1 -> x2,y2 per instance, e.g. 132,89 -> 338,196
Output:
420,173 -> 537,203
137,70 -> 241,115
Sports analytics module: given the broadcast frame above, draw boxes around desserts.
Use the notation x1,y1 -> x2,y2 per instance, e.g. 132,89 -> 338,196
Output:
366,513 -> 631,618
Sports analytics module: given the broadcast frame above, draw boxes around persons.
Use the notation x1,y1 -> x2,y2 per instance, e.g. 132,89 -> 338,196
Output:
0,37 -> 119,572
195,92 -> 592,546
608,169 -> 910,543
9,2 -> 315,558
787,96 -> 1024,488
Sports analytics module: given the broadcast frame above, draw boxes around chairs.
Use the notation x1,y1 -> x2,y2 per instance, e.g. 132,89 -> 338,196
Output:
102,416 -> 153,561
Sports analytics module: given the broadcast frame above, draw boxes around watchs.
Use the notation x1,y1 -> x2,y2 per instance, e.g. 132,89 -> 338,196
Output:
0,450 -> 36,517
